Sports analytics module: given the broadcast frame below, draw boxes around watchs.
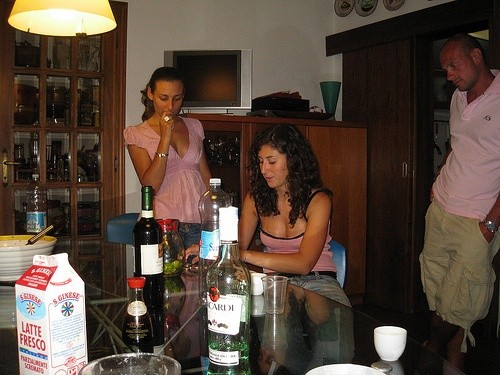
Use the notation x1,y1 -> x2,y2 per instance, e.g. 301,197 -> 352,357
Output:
483,219 -> 498,233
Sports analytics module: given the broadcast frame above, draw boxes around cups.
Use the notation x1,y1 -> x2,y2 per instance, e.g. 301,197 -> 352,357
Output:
319,81 -> 340,121
379,359 -> 404,375
373,326 -> 407,361
78,352 -> 181,375
251,273 -> 267,296
261,275 -> 288,314
261,313 -> 288,351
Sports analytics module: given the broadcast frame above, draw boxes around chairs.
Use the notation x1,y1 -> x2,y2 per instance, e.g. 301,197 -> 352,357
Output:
106,212 -> 138,243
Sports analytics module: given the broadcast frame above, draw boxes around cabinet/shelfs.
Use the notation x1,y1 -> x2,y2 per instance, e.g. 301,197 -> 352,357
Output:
0,0 -> 127,358
179,113 -> 368,304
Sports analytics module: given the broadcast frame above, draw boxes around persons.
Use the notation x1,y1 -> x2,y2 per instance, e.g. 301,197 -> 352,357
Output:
185,123 -> 353,375
163,260 -> 329,375
414,34 -> 500,375
123,66 -> 212,264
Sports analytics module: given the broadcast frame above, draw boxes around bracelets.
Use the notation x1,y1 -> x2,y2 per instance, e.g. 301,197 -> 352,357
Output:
155,152 -> 169,158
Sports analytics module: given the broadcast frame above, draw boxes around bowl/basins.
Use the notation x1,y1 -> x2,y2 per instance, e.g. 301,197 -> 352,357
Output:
0,234 -> 57,281
306,363 -> 386,375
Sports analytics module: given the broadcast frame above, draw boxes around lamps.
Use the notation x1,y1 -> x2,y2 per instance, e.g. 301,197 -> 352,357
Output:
8,0 -> 118,41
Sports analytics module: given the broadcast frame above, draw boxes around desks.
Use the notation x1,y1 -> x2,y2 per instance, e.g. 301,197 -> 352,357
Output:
0,238 -> 470,375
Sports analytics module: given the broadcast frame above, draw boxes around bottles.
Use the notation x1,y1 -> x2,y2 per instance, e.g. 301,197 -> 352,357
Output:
206,207 -> 252,375
133,271 -> 165,357
162,277 -> 186,322
12,84 -> 101,128
12,142 -> 101,183
122,277 -> 155,360
132,185 -> 164,309
198,178 -> 232,298
25,174 -> 47,235
156,218 -> 185,278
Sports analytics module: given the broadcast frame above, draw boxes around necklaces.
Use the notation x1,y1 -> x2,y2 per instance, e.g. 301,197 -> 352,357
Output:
275,191 -> 291,198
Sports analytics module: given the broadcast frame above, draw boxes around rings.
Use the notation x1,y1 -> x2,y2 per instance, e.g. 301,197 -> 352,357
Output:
163,116 -> 169,122
